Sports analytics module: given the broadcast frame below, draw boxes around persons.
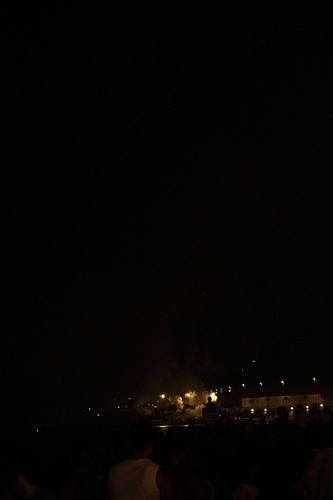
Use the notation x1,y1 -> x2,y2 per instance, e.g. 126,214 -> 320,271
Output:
1,427 -> 333,499
294,403 -> 308,428
322,407 -> 333,424
204,396 -> 217,426
278,406 -> 290,428
310,403 -> 321,426
